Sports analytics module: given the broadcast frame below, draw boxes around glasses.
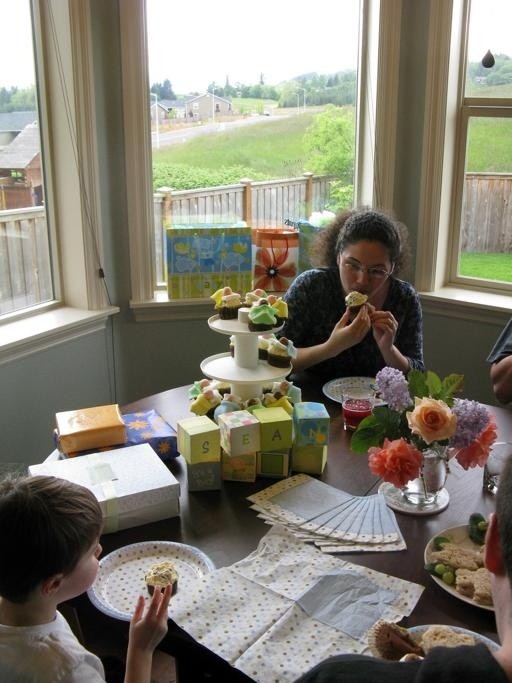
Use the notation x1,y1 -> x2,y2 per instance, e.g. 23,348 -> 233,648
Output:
338,252 -> 395,279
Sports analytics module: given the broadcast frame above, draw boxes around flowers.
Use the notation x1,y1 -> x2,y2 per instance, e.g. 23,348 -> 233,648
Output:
351,366 -> 497,489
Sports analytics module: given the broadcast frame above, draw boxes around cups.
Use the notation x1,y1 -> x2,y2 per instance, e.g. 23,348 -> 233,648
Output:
342,388 -> 375,432
483,443 -> 512,496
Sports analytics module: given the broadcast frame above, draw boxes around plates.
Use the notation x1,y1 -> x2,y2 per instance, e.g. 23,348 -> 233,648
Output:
424,524 -> 497,612
323,376 -> 388,407
378,478 -> 450,516
87,541 -> 216,622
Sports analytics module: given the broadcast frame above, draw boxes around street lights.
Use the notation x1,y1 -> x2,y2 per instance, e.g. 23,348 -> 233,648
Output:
294,91 -> 301,115
148,91 -> 160,149
210,87 -> 217,122
301,86 -> 307,110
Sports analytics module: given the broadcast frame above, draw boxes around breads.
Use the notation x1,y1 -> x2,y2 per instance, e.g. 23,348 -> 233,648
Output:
438,543 -> 493,606
421,625 -> 475,656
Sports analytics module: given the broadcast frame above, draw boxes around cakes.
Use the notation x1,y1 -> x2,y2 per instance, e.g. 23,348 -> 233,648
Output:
144,561 -> 180,598
210,286 -> 289,334
187,376 -> 302,423
344,291 -> 368,316
230,332 -> 298,371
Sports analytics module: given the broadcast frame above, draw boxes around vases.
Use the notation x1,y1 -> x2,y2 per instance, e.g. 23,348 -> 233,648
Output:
398,450 -> 449,505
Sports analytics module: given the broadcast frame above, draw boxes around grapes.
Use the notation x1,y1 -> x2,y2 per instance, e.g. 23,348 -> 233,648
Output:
478,521 -> 489,531
442,571 -> 454,585
433,564 -> 447,575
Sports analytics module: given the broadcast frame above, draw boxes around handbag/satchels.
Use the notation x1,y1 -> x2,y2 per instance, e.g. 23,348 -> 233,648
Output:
251,228 -> 300,292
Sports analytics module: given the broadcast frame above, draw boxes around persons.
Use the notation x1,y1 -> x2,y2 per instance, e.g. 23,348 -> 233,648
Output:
292,452 -> 511,682
0,463 -> 173,683
274,205 -> 425,402
485,318 -> 512,405
